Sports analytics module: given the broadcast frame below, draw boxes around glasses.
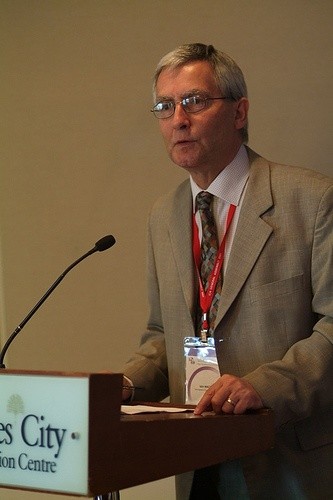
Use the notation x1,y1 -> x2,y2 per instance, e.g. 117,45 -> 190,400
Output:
150,96 -> 237,119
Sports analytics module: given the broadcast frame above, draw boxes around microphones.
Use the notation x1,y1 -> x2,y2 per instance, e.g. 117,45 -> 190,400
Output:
0,235 -> 116,368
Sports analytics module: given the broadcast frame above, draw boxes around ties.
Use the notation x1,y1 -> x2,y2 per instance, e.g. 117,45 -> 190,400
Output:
193,190 -> 223,338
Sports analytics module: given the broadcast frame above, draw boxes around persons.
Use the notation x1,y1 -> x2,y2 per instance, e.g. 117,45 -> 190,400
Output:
95,42 -> 333,500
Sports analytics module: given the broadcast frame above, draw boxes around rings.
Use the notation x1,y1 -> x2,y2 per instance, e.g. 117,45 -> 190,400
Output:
225,397 -> 235,406
